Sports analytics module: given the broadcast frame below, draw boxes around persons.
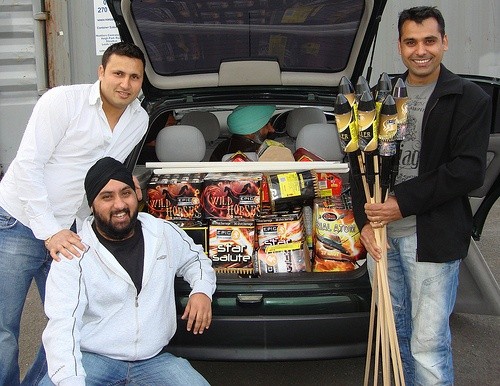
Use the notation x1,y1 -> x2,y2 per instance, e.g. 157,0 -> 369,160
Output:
211,105 -> 277,162
37,157 -> 216,386
0,42 -> 149,385
348,6 -> 492,386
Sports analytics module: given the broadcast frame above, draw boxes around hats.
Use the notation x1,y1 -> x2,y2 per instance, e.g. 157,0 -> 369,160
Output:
84,156 -> 136,208
227,104 -> 276,135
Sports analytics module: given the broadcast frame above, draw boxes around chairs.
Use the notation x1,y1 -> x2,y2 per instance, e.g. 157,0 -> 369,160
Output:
275,107 -> 328,152
180,111 -> 223,161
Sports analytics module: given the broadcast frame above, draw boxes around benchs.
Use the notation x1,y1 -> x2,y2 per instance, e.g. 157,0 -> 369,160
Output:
155,125 -> 346,161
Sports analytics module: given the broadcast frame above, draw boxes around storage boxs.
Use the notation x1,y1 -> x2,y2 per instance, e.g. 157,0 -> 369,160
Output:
146,172 -> 367,275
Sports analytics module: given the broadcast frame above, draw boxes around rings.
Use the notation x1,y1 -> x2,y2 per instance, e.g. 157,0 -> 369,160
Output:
380,222 -> 384,228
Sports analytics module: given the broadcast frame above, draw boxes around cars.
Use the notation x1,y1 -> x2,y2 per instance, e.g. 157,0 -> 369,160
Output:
105,0 -> 500,365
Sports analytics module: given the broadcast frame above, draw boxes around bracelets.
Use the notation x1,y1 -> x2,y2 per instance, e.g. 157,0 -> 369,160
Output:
44,235 -> 52,246
